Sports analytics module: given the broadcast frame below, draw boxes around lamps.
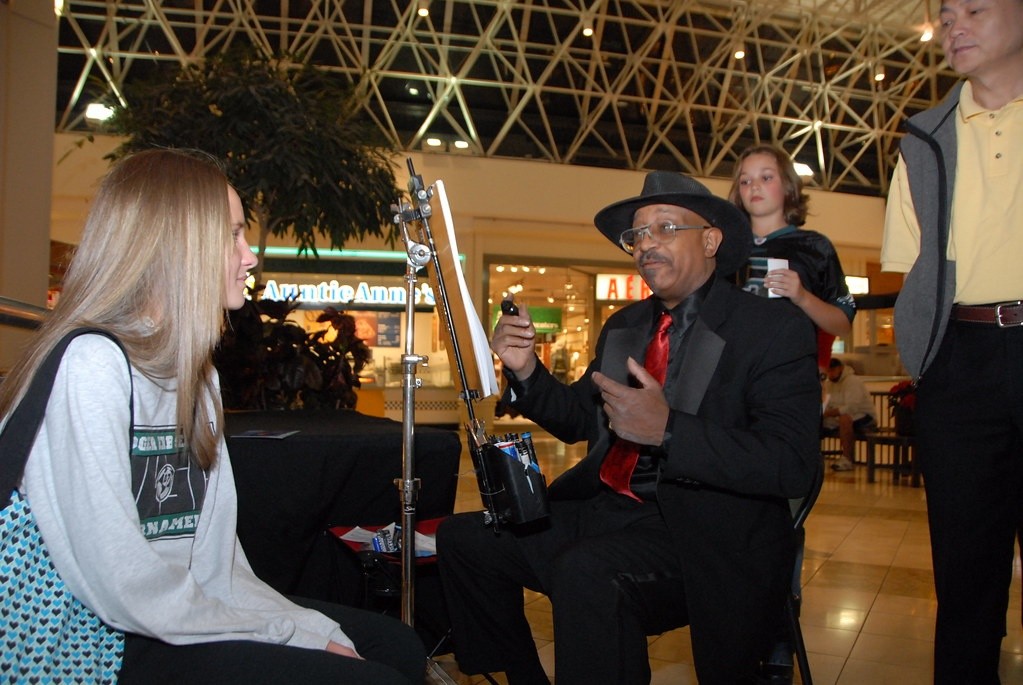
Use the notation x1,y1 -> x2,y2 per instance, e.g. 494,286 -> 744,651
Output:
582,20 -> 594,36
875,62 -> 886,81
418,0 -> 429,16
733,42 -> 747,59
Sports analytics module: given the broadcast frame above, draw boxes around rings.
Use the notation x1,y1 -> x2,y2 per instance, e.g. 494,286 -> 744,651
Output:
608,420 -> 613,429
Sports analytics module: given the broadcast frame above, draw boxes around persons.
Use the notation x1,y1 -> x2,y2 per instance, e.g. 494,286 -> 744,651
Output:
434,170 -> 824,685
821,352 -> 878,471
2,148 -> 428,685
880,0 -> 1023,684
724,144 -> 858,683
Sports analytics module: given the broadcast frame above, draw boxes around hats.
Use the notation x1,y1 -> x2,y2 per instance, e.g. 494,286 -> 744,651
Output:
829,359 -> 840,367
594,172 -> 755,276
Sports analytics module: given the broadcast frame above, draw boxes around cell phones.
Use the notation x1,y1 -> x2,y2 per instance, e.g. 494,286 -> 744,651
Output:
768,259 -> 789,298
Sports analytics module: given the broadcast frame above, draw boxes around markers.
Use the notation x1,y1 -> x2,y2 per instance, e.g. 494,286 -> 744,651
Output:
501,299 -> 519,316
489,432 -> 541,474
372,525 -> 402,551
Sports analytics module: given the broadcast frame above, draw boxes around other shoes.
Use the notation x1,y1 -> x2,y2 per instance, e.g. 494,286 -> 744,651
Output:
831,456 -> 855,471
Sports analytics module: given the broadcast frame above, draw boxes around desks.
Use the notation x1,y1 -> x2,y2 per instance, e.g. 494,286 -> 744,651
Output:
223,410 -> 465,609
866,432 -> 921,486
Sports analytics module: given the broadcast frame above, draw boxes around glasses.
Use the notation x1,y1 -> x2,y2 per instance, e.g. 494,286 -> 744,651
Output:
618,219 -> 713,254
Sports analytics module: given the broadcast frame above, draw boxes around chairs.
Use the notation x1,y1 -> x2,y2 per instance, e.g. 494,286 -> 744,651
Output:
782,455 -> 824,685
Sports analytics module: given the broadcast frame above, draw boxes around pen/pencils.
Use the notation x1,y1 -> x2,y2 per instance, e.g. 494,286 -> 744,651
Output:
463,418 -> 489,446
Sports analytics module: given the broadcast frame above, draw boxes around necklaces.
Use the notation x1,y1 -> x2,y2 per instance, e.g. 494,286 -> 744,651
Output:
129,359 -> 177,392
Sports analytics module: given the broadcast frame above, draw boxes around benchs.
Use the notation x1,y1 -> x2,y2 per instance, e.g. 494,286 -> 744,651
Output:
819,391 -> 893,482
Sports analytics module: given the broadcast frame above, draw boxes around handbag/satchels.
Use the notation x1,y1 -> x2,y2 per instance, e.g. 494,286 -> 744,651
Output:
0,327 -> 133,685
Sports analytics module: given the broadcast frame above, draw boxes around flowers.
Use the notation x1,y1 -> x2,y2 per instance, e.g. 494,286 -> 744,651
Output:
249,295 -> 378,401
888,379 -> 918,421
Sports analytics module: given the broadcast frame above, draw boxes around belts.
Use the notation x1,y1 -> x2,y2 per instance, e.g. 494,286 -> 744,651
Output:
950,301 -> 1023,328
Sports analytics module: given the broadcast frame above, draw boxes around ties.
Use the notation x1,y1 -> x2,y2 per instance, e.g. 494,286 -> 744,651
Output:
599,313 -> 673,505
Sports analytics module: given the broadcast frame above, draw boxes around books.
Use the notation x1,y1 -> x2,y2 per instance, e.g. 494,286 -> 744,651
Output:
230,427 -> 300,439
